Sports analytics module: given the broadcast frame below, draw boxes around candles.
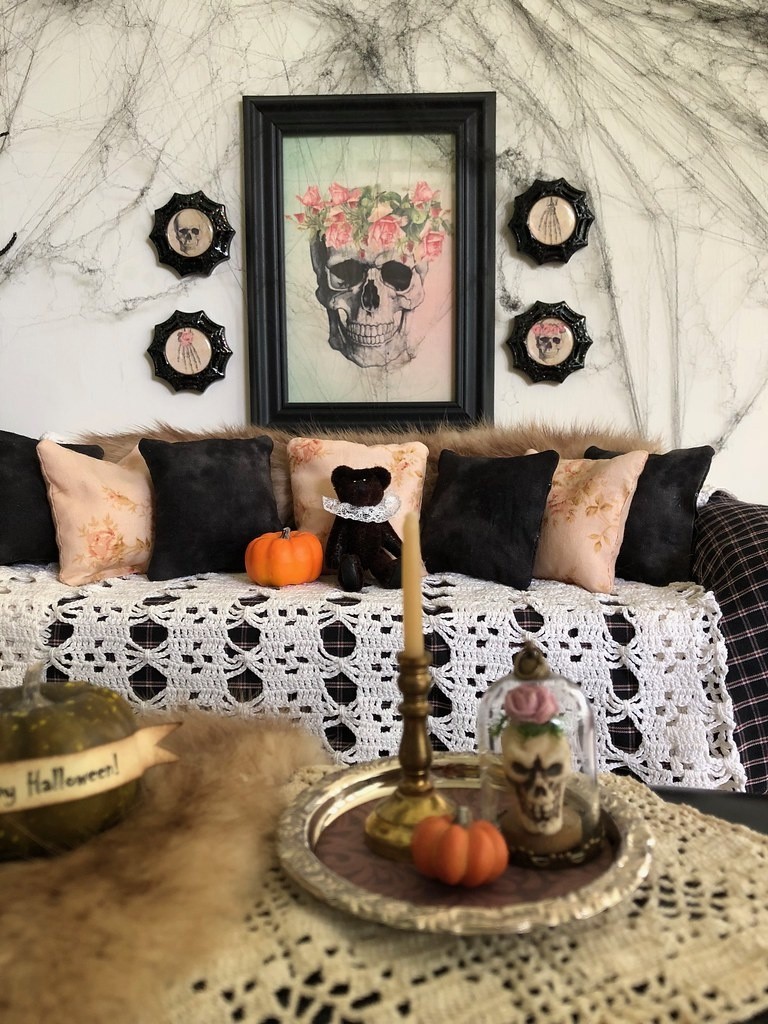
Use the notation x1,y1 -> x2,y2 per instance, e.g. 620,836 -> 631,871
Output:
401,505 -> 426,651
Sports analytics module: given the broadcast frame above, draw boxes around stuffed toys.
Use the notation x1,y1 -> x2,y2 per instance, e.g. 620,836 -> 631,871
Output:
325,465 -> 407,592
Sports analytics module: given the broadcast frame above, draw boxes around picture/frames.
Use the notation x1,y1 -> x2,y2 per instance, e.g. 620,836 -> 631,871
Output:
506,298 -> 592,384
149,188 -> 237,280
506,178 -> 595,265
239,91 -> 496,430
146,309 -> 233,395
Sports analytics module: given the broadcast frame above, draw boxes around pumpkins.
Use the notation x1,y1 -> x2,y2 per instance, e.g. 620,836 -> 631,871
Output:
245,527 -> 323,588
0,660 -> 146,859
410,805 -> 510,889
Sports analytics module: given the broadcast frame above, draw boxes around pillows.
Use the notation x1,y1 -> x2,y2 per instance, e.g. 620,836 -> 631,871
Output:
138,436 -> 283,581
420,449 -> 560,590
286,438 -> 429,579
583,444 -> 715,587
0,432 -> 105,566
523,449 -> 649,592
36,438 -> 156,587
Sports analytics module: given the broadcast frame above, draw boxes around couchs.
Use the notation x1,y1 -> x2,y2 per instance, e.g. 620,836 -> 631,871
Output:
0,422 -> 766,792
